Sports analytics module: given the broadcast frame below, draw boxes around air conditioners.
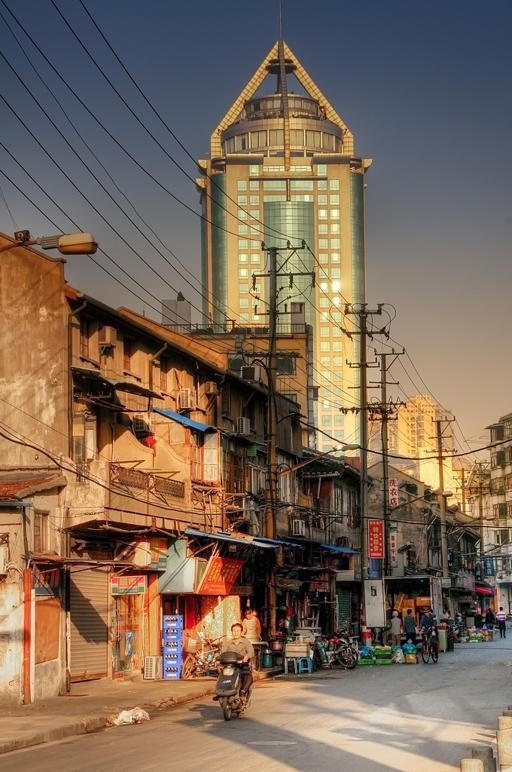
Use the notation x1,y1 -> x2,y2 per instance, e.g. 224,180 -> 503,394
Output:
0,547 -> 10,577
204,380 -> 218,395
235,416 -> 251,434
132,411 -> 151,433
292,518 -> 306,538
111,538 -> 152,568
144,655 -> 163,679
178,388 -> 198,410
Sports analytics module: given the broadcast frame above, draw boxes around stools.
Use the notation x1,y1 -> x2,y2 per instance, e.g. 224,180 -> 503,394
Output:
298,657 -> 314,675
284,656 -> 298,675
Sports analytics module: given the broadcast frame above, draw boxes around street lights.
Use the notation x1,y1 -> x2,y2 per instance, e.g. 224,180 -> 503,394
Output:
381,488 -> 455,578
439,514 -> 496,577
0,231 -> 101,260
267,443 -> 361,639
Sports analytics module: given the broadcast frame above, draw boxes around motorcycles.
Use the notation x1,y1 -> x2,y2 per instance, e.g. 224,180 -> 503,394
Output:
211,653 -> 254,724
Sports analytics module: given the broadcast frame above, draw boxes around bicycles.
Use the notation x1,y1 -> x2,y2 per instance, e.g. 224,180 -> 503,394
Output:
317,629 -> 360,672
417,628 -> 440,665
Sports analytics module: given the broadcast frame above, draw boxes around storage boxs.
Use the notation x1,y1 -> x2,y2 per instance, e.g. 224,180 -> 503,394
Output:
390,591 -> 430,625
285,651 -> 311,657
285,642 -> 310,652
466,628 -> 493,642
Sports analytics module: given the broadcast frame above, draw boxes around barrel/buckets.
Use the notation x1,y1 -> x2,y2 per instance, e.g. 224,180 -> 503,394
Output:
262,653 -> 272,668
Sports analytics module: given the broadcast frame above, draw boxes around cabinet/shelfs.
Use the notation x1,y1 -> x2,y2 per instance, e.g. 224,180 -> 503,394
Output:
438,629 -> 447,652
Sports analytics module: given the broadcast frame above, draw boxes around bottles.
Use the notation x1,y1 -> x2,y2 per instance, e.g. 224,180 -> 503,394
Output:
166,617 -> 178,673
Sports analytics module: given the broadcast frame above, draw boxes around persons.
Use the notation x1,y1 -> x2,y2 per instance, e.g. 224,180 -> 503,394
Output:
213,605 -> 507,701
